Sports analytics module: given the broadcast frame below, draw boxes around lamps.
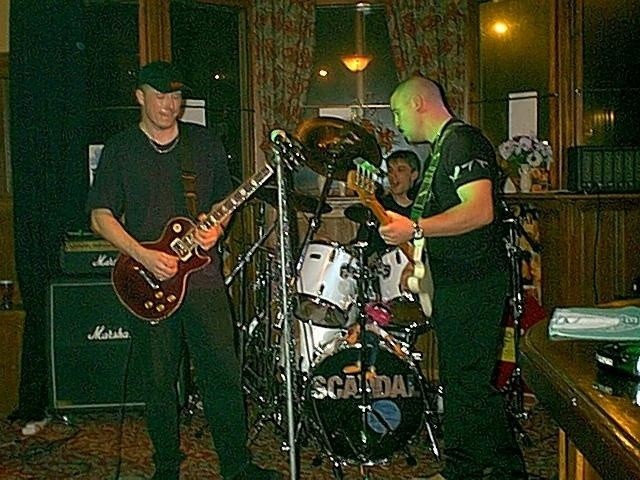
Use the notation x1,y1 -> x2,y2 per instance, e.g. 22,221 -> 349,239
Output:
337,3 -> 375,72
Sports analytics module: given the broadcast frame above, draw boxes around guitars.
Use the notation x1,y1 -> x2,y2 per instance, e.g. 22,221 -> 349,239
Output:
111,138 -> 306,323
347,156 -> 434,318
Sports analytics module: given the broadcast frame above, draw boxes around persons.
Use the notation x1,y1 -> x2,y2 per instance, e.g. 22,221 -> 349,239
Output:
357,150 -> 422,254
86,60 -> 283,478
388,74 -> 528,480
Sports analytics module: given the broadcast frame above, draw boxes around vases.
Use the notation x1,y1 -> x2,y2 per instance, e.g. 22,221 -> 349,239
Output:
517,164 -> 538,193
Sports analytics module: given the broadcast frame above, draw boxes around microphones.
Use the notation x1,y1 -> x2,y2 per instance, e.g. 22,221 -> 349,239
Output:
271,128 -> 308,166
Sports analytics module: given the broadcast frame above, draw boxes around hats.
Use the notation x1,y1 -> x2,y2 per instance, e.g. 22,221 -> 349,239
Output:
139,60 -> 193,94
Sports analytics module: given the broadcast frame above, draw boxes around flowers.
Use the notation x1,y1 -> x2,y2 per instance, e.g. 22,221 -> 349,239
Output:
498,133 -> 556,176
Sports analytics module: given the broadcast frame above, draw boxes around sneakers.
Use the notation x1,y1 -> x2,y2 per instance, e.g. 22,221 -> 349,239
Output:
150,467 -> 179,480
231,464 -> 284,479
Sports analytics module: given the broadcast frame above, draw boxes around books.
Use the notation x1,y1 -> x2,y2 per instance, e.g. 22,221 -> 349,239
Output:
567,145 -> 640,194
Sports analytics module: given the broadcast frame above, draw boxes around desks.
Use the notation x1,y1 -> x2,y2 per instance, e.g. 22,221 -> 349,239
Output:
521,297 -> 639,480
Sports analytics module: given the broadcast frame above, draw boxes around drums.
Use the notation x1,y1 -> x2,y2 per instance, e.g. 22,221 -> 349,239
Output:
293,239 -> 360,329
293,300 -> 356,384
308,331 -> 425,465
377,248 -> 415,303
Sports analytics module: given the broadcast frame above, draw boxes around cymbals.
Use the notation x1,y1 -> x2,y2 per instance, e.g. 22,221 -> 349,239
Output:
255,184 -> 333,214
344,203 -> 367,224
291,116 -> 382,182
